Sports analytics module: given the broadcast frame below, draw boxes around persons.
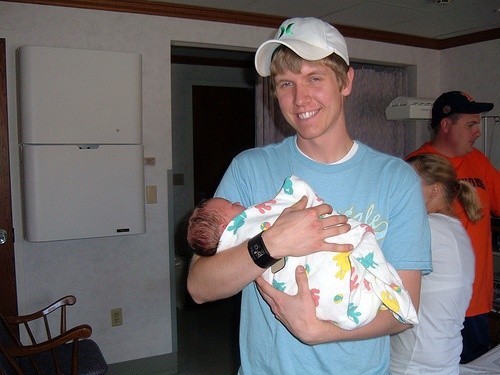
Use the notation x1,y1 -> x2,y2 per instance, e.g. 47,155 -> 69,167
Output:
403,90 -> 500,365
187,16 -> 433,375
187,174 -> 420,331
389,152 -> 486,375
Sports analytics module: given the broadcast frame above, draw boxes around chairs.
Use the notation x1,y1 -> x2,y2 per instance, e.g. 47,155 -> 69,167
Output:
0,295 -> 108,375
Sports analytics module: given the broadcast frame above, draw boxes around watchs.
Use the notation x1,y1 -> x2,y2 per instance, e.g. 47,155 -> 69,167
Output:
247,230 -> 286,274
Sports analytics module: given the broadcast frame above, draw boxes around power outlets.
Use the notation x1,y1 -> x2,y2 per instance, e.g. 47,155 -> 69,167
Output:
111,308 -> 123,327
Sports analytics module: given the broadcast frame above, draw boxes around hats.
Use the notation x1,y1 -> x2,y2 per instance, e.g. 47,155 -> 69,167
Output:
431,91 -> 494,127
255,16 -> 350,77
